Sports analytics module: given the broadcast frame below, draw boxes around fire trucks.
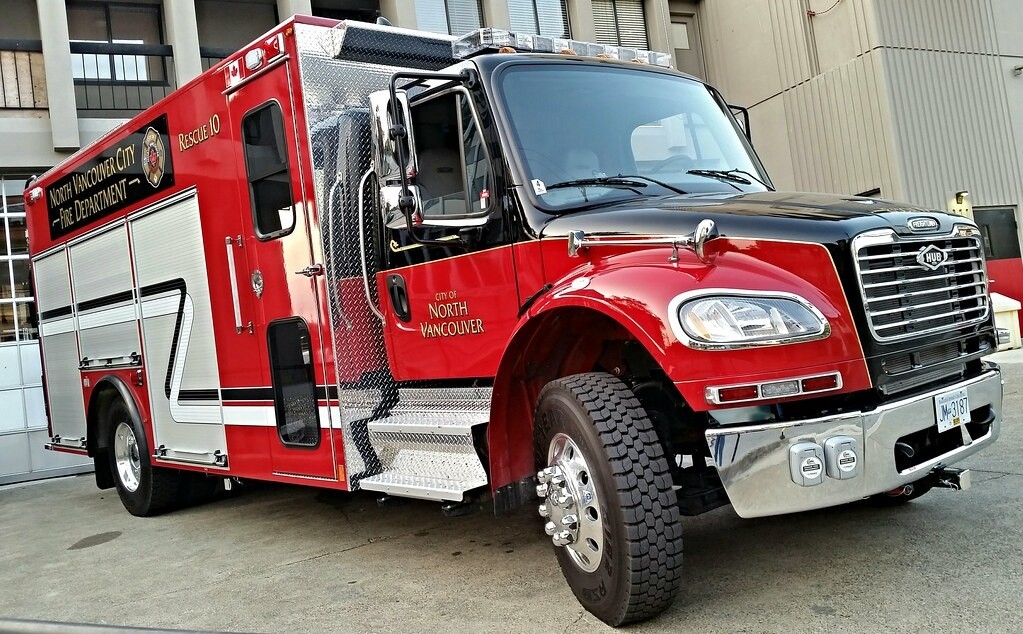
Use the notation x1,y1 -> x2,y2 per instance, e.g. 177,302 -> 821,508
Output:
20,15 -> 1005,627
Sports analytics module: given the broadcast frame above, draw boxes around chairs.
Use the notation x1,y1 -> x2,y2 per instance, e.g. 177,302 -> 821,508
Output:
557,136 -> 600,181
417,149 -> 465,213
